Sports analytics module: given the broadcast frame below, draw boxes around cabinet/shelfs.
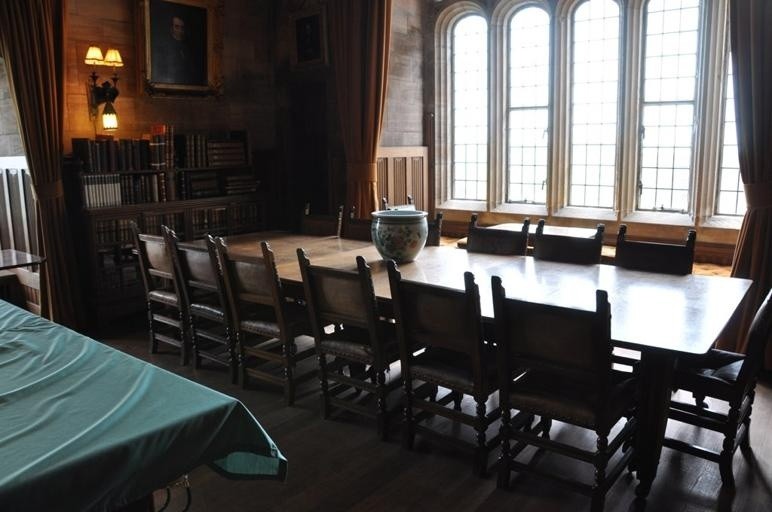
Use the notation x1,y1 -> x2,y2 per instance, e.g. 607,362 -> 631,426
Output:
69,165 -> 274,337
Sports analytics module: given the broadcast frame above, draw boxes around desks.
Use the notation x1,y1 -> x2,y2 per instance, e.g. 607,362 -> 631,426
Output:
0,297 -> 290,512
0,241 -> 47,280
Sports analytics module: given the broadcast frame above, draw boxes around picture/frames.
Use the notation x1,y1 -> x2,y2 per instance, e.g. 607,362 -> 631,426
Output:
286,4 -> 330,70
137,1 -> 226,103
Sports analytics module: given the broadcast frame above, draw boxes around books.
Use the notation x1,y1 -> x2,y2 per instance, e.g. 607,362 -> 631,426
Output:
71,122 -> 289,295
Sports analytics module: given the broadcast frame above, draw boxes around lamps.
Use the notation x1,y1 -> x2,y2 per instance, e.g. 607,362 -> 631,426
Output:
82,43 -> 128,134
